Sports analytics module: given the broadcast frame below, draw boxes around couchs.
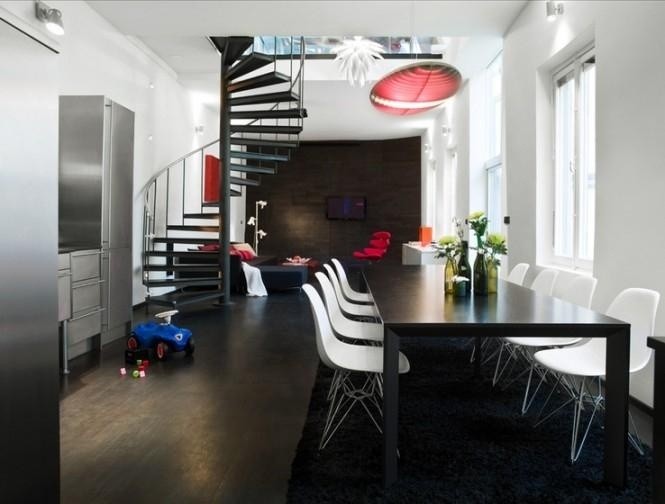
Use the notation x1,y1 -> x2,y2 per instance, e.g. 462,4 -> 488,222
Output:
175,240 -> 308,294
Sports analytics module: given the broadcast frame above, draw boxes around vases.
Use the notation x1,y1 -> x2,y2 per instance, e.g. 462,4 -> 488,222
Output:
454,241 -> 471,296
444,257 -> 458,295
474,248 -> 488,297
488,259 -> 497,295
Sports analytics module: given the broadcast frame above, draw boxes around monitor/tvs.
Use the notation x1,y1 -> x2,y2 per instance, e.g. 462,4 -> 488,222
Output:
325,195 -> 367,222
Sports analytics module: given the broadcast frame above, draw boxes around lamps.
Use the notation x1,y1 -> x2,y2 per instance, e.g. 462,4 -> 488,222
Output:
369,61 -> 464,118
545,1 -> 564,17
35,0 -> 64,36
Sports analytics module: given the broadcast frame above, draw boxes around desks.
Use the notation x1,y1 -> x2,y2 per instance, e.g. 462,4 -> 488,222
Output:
358,264 -> 631,495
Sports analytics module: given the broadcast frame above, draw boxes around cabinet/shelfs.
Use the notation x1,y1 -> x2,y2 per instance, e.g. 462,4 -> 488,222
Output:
401,242 -> 447,264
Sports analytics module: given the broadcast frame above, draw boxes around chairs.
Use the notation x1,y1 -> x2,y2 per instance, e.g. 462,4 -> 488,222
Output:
470,262 -> 660,466
300,258 -> 410,458
301,283 -> 410,458
351,231 -> 391,261
532,286 -> 660,466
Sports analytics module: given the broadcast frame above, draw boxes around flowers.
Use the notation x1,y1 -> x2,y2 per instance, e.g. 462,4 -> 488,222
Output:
430,236 -> 464,259
485,233 -> 508,260
451,215 -> 465,240
468,211 -> 488,249
